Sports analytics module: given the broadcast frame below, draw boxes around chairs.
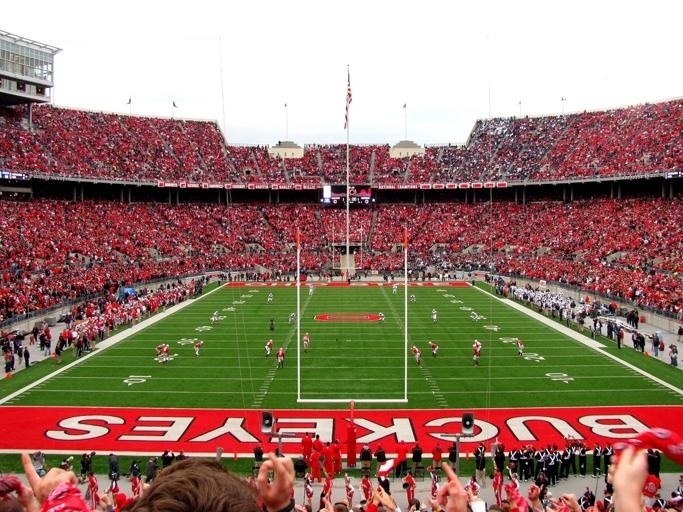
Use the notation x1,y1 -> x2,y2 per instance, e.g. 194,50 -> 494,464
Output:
401,467 -> 412,477
253,467 -> 260,478
435,467 -> 441,480
414,467 -> 425,481
361,467 -> 371,480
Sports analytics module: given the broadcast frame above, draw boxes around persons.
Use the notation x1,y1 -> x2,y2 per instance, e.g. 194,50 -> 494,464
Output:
0,102 -> 683,377
1,417 -> 683,512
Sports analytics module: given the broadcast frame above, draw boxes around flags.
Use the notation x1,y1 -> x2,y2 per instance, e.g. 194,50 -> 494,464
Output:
342,67 -> 353,130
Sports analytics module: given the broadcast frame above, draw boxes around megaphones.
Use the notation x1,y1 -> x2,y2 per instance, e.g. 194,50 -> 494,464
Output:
462,413 -> 474,434
262,412 -> 275,433
